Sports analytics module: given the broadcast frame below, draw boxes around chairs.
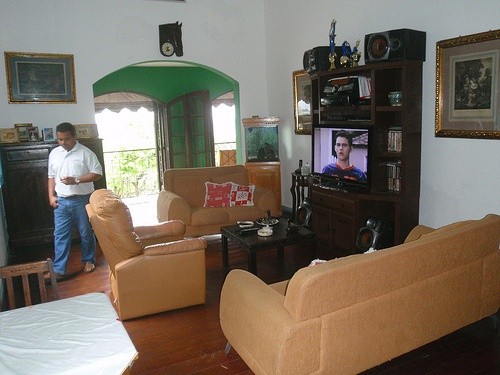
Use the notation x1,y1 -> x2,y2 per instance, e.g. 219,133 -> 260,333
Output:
0,258 -> 60,310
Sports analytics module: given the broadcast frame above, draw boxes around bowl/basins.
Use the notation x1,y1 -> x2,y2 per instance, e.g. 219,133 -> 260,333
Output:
388,91 -> 404,106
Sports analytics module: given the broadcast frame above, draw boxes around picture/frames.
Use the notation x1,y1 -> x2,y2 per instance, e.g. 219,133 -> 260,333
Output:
4,51 -> 76,105
292,69 -> 312,135
435,29 -> 500,141
14,124 -> 54,142
73,124 -> 97,139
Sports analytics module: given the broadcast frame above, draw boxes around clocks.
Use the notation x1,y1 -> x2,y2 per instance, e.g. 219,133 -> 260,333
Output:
158,21 -> 184,58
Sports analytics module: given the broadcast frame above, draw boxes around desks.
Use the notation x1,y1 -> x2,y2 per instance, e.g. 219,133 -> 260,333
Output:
0,290 -> 139,375
290,171 -> 309,221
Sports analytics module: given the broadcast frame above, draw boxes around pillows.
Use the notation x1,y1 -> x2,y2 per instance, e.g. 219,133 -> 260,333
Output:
204,181 -> 255,208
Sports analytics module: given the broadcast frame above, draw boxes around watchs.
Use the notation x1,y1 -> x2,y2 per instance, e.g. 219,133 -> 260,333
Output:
75,177 -> 80,185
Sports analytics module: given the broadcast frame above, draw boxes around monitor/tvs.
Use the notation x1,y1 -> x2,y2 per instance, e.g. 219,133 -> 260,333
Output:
310,125 -> 372,193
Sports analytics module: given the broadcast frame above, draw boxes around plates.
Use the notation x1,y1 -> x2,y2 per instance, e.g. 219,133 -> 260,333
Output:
257,218 -> 279,225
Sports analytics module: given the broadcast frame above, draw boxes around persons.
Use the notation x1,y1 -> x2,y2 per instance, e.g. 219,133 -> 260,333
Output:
42,122 -> 103,280
321,130 -> 367,182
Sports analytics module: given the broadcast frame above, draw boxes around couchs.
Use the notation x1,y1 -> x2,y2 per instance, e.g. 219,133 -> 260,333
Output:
85,188 -> 206,320
219,214 -> 500,375
156,166 -> 276,238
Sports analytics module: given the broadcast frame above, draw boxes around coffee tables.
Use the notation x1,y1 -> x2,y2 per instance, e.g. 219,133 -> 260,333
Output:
221,217 -> 315,278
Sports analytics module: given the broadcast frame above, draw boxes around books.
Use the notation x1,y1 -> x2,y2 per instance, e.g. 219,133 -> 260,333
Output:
387,130 -> 402,152
386,160 -> 401,192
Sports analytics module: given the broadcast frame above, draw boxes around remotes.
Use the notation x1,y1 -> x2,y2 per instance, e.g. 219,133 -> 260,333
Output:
239,228 -> 260,235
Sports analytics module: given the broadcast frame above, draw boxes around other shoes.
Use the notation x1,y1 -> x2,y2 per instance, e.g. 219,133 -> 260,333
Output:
84,262 -> 95,273
43,272 -> 65,282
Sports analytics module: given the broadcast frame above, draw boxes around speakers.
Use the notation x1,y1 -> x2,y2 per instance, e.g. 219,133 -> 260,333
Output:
355,217 -> 394,253
303,46 -> 350,74
364,28 -> 427,64
296,198 -> 313,229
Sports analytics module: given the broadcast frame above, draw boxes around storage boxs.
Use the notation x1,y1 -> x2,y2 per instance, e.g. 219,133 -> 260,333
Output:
242,116 -> 280,163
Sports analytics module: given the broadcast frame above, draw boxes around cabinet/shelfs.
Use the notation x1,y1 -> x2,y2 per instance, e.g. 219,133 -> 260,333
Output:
0,138 -> 107,259
309,57 -> 423,259
245,162 -> 283,218
